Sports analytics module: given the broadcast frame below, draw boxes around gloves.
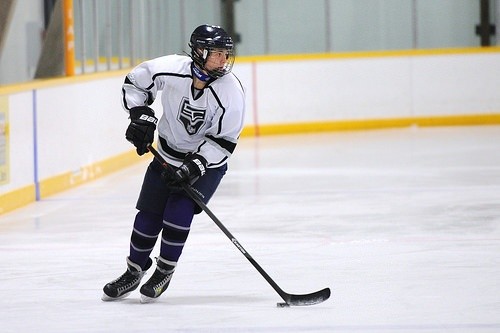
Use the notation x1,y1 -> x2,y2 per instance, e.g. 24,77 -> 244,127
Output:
124,106 -> 157,156
170,151 -> 206,196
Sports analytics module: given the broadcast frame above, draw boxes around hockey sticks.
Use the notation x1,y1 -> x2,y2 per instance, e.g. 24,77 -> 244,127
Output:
146,143 -> 331,306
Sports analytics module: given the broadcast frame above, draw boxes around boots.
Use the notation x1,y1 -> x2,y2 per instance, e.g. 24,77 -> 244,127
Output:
139,256 -> 178,302
101,255 -> 153,301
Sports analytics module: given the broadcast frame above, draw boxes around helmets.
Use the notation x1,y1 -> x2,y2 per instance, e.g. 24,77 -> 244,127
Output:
187,25 -> 235,78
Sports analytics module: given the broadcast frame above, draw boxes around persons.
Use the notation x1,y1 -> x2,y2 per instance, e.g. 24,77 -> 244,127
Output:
102,25 -> 246,303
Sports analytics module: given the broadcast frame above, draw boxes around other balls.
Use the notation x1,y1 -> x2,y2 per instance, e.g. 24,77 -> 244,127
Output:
277,302 -> 289,307
